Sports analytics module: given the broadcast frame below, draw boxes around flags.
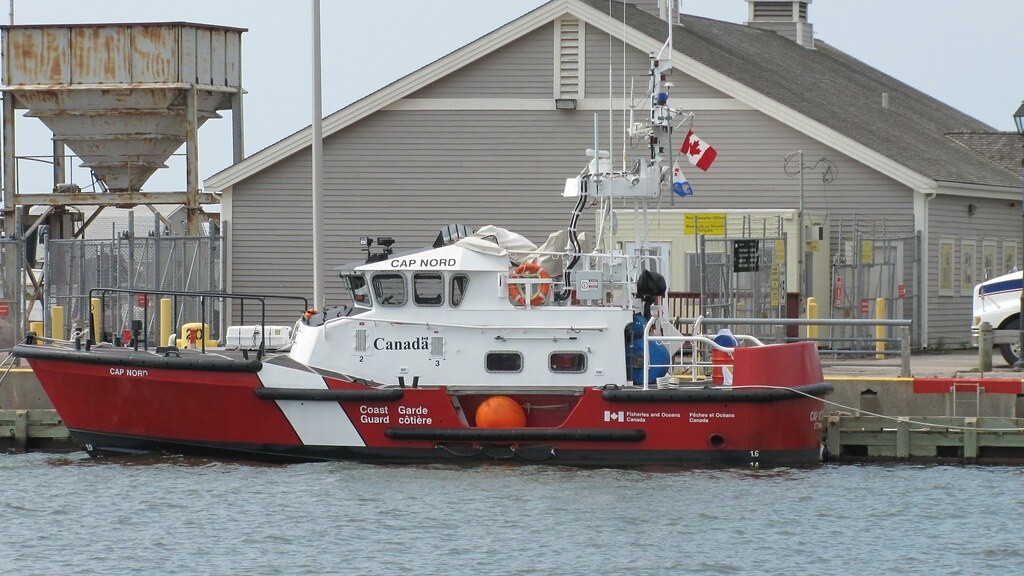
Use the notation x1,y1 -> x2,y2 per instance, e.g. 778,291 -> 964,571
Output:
670,162 -> 693,197
680,131 -> 717,171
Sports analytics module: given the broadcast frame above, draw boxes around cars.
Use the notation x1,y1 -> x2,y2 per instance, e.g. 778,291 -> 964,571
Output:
971,266 -> 1024,367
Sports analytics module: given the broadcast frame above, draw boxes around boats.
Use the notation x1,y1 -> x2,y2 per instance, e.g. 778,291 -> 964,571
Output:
9,0 -> 834,469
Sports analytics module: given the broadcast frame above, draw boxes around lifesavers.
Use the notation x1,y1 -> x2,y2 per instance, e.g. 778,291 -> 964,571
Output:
509,265 -> 551,306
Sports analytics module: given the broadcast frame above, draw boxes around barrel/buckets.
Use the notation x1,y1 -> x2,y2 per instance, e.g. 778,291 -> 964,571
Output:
712,348 -> 734,387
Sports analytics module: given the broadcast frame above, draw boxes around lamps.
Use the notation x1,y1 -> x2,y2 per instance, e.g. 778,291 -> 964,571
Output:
555,98 -> 577,110
969,204 -> 978,215
360,236 -> 397,264
